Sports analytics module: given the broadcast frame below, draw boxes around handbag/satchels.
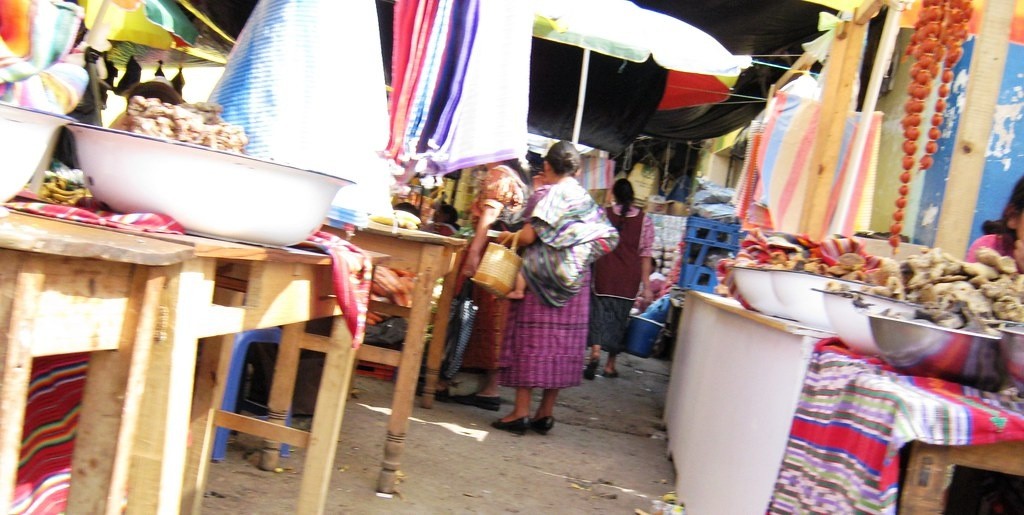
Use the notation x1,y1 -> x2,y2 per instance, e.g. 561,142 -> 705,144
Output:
438,277 -> 480,382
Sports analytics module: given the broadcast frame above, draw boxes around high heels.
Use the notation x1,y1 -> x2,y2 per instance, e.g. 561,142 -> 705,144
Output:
528,416 -> 555,435
491,415 -> 530,435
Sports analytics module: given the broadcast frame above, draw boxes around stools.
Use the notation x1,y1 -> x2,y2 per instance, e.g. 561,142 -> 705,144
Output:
211,328 -> 292,462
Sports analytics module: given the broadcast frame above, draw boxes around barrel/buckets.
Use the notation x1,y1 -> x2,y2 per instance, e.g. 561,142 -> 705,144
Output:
626,315 -> 665,357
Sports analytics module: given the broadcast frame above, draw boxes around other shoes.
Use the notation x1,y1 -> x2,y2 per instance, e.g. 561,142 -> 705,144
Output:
602,370 -> 619,378
583,358 -> 599,380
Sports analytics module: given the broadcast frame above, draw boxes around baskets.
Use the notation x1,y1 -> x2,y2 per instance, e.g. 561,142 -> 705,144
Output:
470,231 -> 525,300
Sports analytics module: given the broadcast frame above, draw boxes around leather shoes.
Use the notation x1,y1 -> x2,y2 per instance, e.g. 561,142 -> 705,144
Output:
452,391 -> 501,412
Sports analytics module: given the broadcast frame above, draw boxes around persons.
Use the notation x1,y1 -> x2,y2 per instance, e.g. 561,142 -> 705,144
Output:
109,80 -> 186,130
368,142 -> 655,437
964,174 -> 1024,277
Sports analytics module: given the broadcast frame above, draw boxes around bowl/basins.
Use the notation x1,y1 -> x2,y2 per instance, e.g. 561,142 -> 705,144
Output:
1,102 -> 79,203
730,268 -> 1023,392
64,122 -> 356,247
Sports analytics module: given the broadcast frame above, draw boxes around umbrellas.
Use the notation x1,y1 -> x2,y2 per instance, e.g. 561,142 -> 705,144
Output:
532,0 -> 742,141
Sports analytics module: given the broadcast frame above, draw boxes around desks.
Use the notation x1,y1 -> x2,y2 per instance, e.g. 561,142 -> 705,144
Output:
259,221 -> 467,496
0,209 -> 390,515
